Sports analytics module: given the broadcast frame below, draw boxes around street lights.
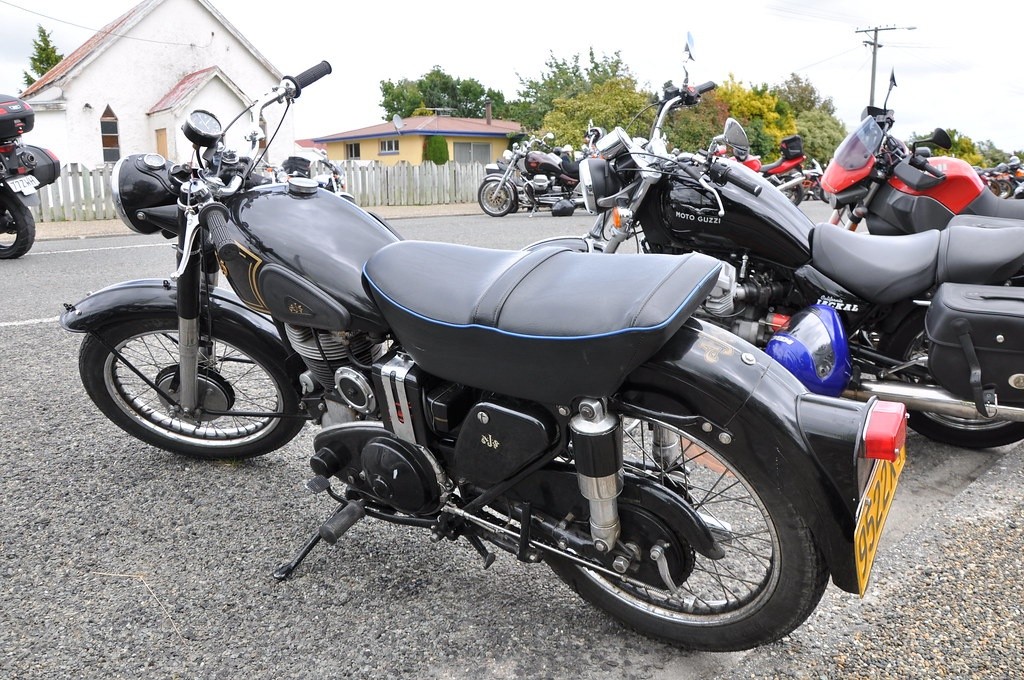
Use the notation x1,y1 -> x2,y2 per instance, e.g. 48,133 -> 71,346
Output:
855,24 -> 917,106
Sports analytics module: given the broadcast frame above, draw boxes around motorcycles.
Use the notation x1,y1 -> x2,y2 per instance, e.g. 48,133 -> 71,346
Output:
974,166 -> 1000,198
803,158 -> 821,201
1014,182 -> 1024,199
59,60 -> 907,653
519,32 -> 1024,449
0,94 -> 61,260
477,133 -> 583,217
993,163 -> 1012,199
1009,156 -> 1024,180
712,135 -> 805,207
821,66 -> 1024,235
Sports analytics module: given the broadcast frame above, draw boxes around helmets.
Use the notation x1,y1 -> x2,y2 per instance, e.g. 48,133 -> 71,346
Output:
551,198 -> 574,217
766,304 -> 853,397
